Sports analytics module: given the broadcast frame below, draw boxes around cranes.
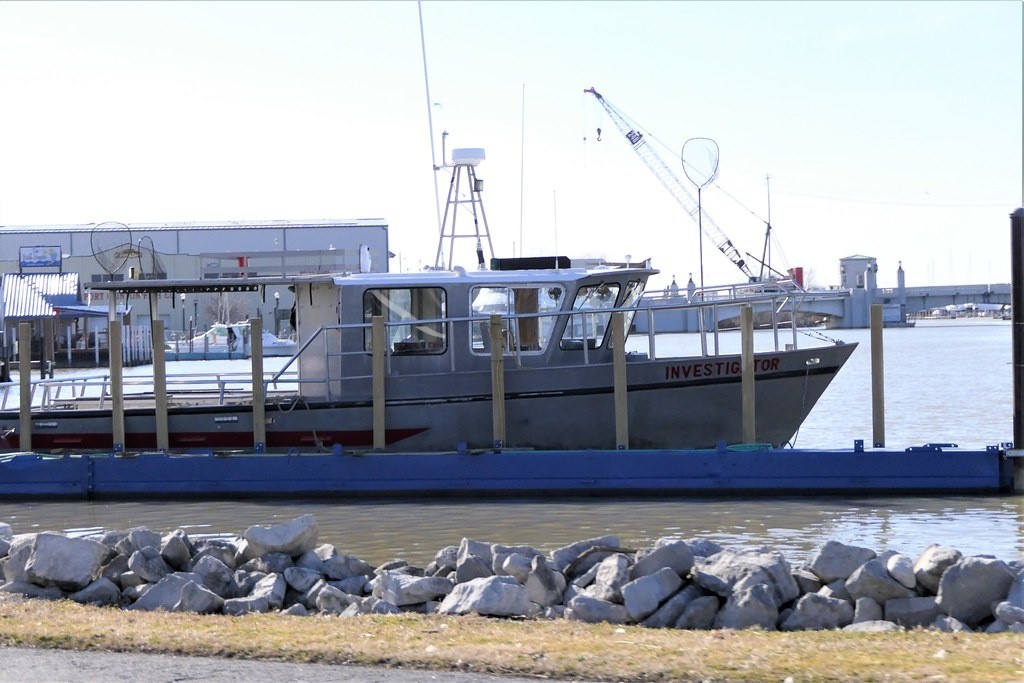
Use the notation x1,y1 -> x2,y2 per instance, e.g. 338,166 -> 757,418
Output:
581,88 -> 798,296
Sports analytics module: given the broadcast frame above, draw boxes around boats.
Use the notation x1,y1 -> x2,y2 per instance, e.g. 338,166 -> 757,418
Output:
0,134 -> 860,451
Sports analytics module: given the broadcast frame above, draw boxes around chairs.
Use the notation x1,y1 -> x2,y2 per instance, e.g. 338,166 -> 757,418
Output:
480,323 -> 491,353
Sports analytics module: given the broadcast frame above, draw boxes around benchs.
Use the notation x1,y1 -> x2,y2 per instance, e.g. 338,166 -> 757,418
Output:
51,394 -> 253,410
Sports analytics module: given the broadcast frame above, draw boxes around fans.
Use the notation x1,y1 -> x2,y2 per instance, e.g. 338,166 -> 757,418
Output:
548,287 -> 562,301
595,286 -> 611,302
